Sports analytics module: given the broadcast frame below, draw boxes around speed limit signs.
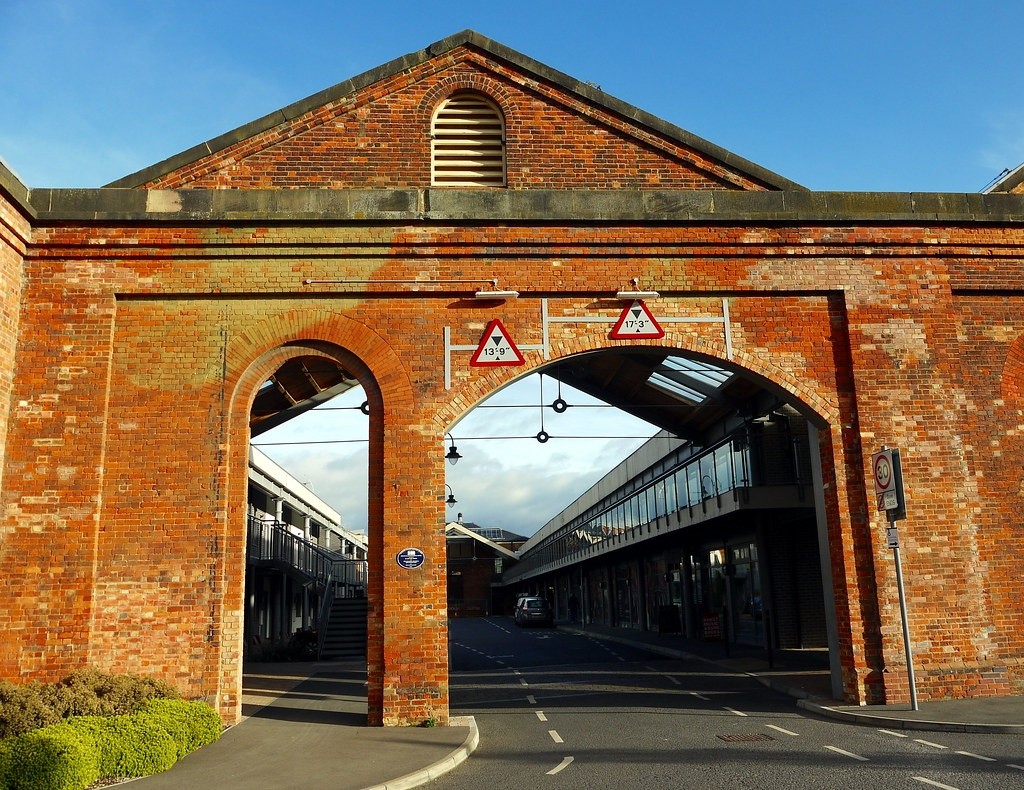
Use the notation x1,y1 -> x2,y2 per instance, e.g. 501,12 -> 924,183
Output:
875,455 -> 891,488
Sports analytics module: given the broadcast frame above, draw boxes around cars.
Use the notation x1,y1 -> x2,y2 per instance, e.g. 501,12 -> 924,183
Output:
513,596 -> 554,629
